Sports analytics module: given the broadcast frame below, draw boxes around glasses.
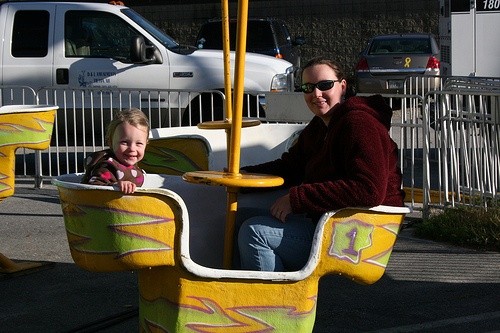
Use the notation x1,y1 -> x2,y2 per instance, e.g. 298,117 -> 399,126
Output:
300,80 -> 340,93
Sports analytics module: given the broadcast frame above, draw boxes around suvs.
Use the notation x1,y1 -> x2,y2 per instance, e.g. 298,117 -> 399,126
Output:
195,16 -> 294,63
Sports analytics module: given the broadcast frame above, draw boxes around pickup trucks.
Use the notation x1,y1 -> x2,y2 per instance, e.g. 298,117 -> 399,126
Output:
0,1 -> 295,171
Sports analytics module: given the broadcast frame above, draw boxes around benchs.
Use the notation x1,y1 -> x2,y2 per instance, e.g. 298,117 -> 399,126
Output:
51,169 -> 411,287
0,103 -> 59,152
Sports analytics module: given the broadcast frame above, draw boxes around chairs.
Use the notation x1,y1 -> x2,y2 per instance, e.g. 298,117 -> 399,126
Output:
377,45 -> 393,53
65,24 -> 77,56
74,27 -> 90,57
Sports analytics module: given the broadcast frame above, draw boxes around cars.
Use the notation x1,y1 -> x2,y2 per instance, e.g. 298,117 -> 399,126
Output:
354,32 -> 440,110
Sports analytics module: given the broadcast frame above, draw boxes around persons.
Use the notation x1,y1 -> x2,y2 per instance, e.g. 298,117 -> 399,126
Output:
80,108 -> 150,194
223,55 -> 404,271
